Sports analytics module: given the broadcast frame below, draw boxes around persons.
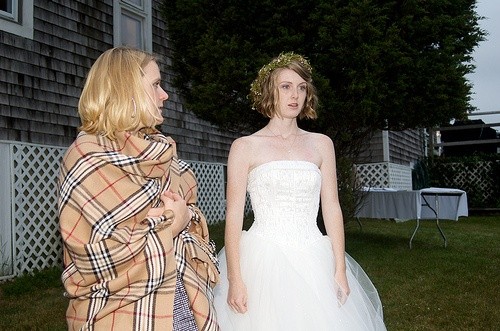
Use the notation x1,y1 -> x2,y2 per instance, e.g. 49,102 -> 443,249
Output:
58,48 -> 221,331
213,50 -> 387,330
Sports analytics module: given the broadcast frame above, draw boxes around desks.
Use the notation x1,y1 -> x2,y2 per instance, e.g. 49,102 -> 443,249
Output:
354,186 -> 468,252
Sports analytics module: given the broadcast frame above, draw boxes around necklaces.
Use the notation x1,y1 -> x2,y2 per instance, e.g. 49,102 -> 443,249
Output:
266,125 -> 299,156
